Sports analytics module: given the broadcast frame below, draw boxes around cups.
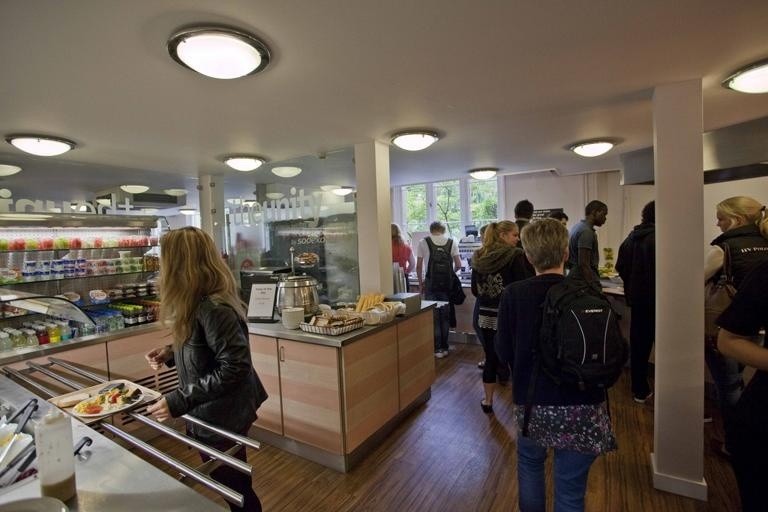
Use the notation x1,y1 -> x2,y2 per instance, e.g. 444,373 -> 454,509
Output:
1,235 -> 159,251
462,234 -> 475,242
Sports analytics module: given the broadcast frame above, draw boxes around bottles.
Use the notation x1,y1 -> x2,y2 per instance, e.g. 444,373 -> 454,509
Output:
0,319 -> 73,353
107,296 -> 162,325
71,308 -> 125,339
35,401 -> 77,501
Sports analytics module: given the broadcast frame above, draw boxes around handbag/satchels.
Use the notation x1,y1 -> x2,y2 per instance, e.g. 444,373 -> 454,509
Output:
704,278 -> 737,338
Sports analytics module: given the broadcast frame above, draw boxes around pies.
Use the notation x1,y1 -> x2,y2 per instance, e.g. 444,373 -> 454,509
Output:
364,303 -> 394,325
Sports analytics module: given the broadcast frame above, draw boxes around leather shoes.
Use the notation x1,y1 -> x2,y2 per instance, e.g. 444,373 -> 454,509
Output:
481,399 -> 492,414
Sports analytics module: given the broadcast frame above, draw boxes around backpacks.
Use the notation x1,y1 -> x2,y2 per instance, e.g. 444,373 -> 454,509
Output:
425,237 -> 453,291
540,280 -> 624,390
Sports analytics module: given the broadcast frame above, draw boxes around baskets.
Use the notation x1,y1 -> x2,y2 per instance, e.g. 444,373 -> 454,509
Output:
323,308 -> 396,325
299,318 -> 365,336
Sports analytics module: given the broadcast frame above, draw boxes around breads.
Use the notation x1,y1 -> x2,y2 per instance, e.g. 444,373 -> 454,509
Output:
356,295 -> 384,313
309,314 -> 361,328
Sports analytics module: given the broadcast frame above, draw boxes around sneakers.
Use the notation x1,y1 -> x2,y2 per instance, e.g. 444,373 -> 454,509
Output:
434,349 -> 448,359
704,414 -> 713,422
711,439 -> 730,459
635,392 -> 653,402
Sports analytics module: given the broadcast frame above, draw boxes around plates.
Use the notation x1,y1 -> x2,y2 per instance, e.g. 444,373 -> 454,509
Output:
281,308 -> 304,329
74,391 -> 144,417
293,252 -> 321,269
64,292 -> 80,302
320,186 -> 345,203
89,290 -> 106,299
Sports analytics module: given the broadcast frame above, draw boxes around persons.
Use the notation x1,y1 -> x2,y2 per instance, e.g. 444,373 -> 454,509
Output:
470,221 -> 535,415
704,196 -> 767,453
716,265 -> 764,512
416,221 -> 461,357
515,199 -> 609,293
614,202 -> 652,407
141,225 -> 268,512
467,226 -> 493,369
495,220 -> 632,512
390,224 -> 416,294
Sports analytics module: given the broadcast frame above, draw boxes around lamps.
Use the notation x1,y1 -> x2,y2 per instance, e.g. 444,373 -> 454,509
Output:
468,167 -> 497,180
6,135 -> 77,158
389,129 -> 438,153
223,155 -> 267,172
721,58 -> 768,94
569,141 -> 615,158
166,25 -> 272,81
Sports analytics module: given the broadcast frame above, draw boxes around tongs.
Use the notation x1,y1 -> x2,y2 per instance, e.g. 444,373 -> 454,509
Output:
0,397 -> 41,488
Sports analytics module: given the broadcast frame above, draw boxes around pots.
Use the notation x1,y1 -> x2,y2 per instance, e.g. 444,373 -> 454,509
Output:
275,274 -> 319,318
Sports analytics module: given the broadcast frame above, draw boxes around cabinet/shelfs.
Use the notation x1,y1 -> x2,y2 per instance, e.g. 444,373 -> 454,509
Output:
0,219 -> 164,363
249,335 -> 343,457
